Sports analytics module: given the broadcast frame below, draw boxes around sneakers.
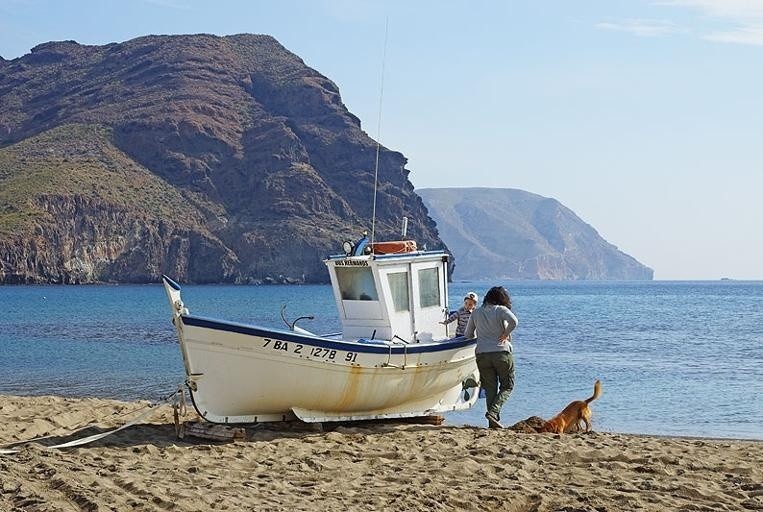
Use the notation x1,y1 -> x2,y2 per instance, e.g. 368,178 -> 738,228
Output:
486,411 -> 503,428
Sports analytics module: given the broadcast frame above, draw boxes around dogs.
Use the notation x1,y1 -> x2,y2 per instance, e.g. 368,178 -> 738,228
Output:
535,379 -> 600,433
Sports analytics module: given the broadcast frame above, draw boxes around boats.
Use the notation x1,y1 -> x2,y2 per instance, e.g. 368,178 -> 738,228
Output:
162,217 -> 482,426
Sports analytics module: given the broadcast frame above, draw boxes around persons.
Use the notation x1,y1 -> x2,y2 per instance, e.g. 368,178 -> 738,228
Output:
464,286 -> 519,429
439,292 -> 479,338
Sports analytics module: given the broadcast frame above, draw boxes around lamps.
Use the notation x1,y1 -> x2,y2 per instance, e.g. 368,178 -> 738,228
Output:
342,240 -> 356,256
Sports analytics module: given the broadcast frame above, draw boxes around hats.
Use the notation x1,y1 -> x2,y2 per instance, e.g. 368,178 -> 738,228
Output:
465,292 -> 478,303
